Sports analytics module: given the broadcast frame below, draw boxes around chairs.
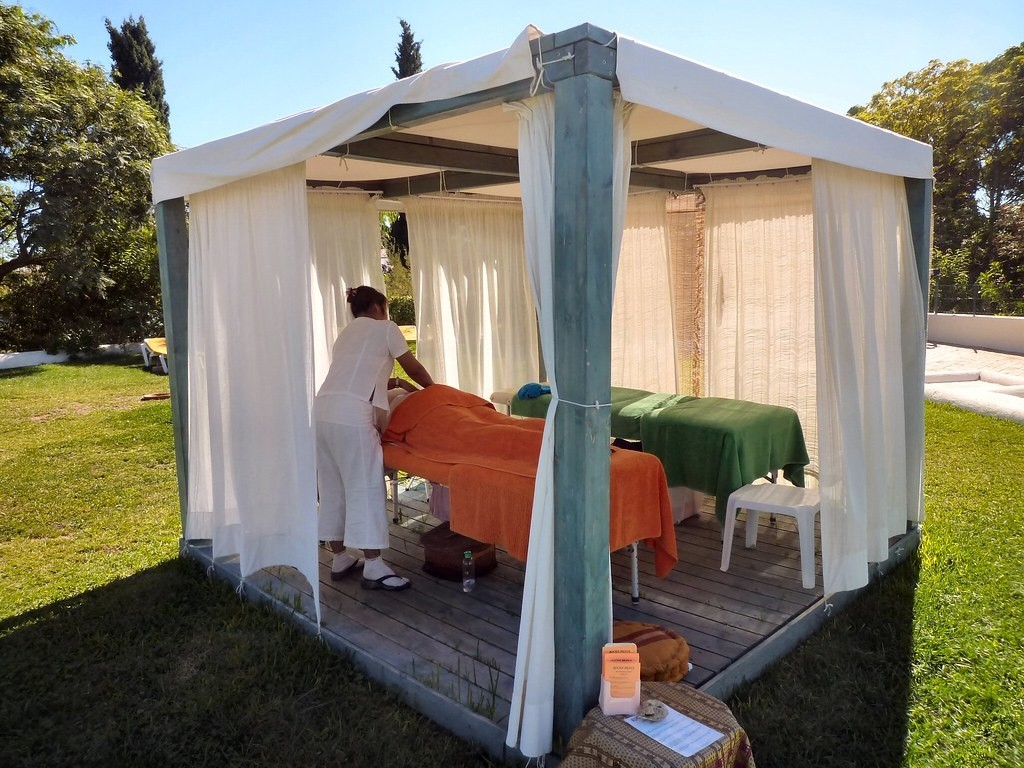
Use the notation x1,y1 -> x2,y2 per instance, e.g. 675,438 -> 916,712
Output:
140,337 -> 206,374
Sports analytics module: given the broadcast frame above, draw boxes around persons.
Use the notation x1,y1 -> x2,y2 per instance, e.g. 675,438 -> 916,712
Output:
312,284 -> 437,592
365,378 -> 615,468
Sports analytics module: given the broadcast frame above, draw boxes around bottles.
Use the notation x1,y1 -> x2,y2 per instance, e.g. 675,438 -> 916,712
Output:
462,551 -> 476,593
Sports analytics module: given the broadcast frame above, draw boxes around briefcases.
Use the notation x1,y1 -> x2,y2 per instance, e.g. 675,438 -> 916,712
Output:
419,521 -> 498,583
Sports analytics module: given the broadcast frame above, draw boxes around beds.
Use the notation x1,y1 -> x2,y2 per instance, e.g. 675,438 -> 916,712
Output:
376,388 -> 679,604
491,382 -> 810,551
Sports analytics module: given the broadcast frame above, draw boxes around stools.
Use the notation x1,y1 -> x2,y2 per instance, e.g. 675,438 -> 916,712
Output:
720,483 -> 822,589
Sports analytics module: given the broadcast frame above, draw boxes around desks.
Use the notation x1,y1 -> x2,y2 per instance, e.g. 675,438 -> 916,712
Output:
559,681 -> 756,768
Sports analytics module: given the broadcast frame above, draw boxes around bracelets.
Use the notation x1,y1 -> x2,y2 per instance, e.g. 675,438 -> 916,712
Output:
395,376 -> 400,387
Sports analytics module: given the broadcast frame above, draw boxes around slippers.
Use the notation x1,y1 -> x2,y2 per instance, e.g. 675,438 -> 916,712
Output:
331,558 -> 365,581
360,572 -> 411,592
